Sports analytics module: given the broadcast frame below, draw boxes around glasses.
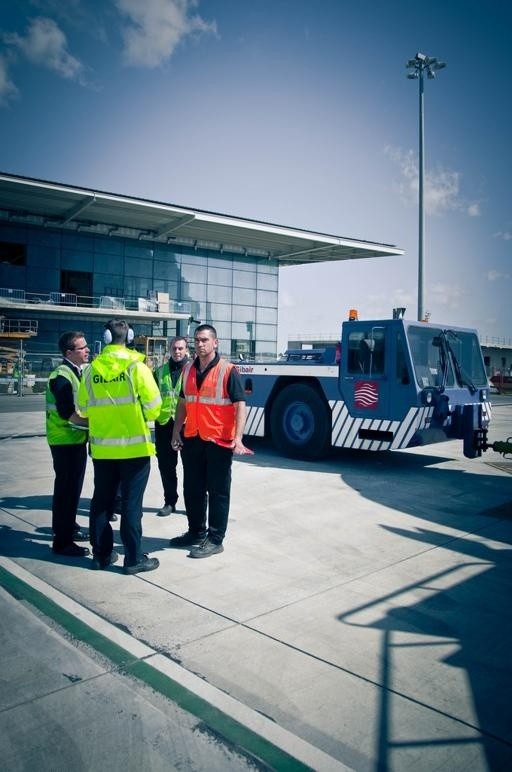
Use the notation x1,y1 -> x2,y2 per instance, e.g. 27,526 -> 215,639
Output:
77,345 -> 88,352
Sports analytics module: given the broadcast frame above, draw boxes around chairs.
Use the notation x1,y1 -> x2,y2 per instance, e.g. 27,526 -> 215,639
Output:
356,337 -> 382,374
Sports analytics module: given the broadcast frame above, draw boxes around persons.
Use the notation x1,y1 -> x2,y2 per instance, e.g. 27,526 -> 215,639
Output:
73,318 -> 162,574
171,323 -> 249,558
45,331 -> 92,555
152,334 -> 197,516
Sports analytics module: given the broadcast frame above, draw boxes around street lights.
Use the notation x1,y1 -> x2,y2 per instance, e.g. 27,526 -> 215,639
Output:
406,51 -> 448,322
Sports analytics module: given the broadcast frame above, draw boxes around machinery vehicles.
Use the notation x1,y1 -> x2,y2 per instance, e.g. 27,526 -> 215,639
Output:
230,307 -> 493,461
131,335 -> 169,372
28,357 -> 64,393
0,317 -> 38,377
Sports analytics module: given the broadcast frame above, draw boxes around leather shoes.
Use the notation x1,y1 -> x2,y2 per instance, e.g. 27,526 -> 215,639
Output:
158,504 -> 175,516
190,538 -> 224,558
54,542 -> 90,556
91,551 -> 118,570
169,533 -> 206,547
72,531 -> 90,541
109,515 -> 117,521
122,553 -> 159,575
